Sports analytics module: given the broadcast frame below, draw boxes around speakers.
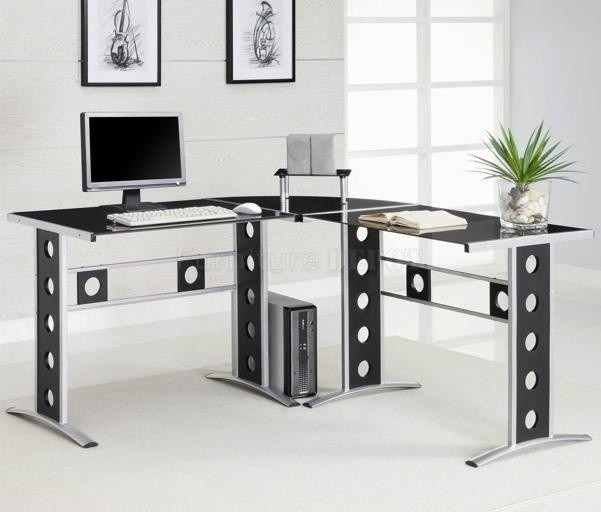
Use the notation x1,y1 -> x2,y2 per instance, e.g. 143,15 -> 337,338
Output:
287,135 -> 310,175
310,134 -> 345,176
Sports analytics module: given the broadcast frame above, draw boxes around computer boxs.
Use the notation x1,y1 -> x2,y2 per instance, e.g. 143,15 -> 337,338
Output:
267,288 -> 318,401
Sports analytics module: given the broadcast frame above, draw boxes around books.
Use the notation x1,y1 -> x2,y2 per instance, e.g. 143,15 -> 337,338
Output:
358,208 -> 469,230
359,220 -> 467,236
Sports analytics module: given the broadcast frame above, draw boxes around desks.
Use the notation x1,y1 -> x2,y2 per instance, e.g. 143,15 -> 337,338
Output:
5,199 -> 301,447
299,202 -> 593,468
215,196 -> 420,407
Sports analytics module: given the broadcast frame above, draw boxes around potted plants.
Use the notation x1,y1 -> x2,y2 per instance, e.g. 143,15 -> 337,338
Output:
466,116 -> 588,231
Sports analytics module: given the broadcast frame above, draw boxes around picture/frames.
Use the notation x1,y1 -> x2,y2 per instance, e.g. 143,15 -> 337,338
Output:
80,0 -> 161,87
224,0 -> 296,84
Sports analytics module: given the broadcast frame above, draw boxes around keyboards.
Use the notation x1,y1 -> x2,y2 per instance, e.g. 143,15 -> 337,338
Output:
105,204 -> 238,228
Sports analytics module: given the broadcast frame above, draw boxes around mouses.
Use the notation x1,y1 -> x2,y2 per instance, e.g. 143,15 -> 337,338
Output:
233,201 -> 264,216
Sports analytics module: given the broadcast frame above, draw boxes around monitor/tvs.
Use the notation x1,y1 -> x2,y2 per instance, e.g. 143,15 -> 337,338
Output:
80,110 -> 187,213
